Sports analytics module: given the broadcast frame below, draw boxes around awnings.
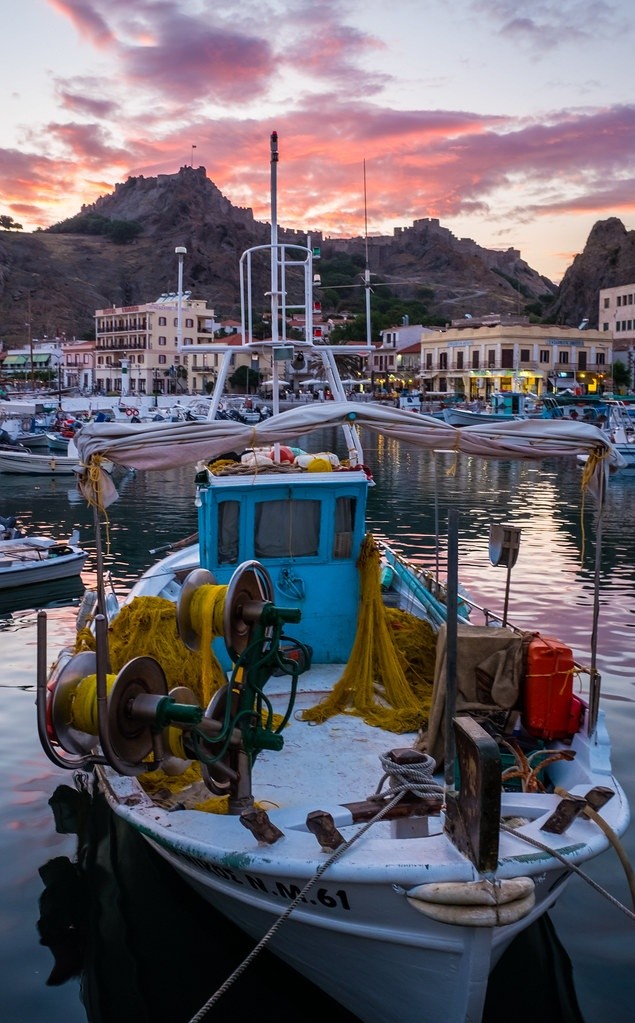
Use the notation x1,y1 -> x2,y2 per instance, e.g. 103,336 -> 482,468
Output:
548,378 -> 579,388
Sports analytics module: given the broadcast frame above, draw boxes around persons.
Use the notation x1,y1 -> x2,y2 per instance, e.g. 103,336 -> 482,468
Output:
0,387 -> 7,400
392,389 -> 397,405
346,389 -> 355,397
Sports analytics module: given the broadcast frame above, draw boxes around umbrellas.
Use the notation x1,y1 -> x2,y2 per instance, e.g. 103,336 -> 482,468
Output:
342,379 -> 380,394
299,379 -> 329,393
262,380 -> 290,386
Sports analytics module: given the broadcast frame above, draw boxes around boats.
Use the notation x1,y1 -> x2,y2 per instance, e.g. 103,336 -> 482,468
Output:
0,369 -> 634,478
0,529 -> 91,591
32,118 -> 634,1023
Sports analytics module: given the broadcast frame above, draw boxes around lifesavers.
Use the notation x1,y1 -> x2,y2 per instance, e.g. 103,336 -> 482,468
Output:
133,410 -> 139,416
126,409 -> 132,416
